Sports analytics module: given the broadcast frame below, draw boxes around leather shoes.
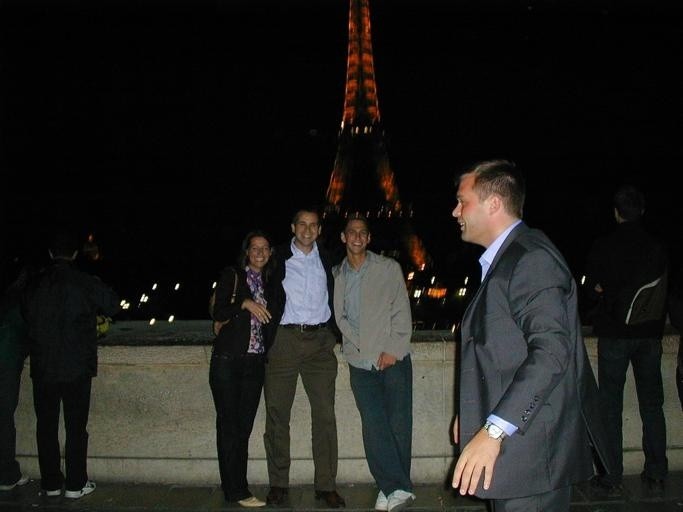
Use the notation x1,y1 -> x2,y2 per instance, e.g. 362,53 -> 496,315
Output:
314,489 -> 346,509
238,497 -> 265,508
266,487 -> 290,507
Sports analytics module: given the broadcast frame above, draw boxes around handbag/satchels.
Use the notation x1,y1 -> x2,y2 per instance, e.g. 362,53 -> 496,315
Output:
206,265 -> 239,334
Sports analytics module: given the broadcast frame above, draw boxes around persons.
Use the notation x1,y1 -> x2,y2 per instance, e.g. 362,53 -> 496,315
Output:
207,231 -> 273,509
447,154 -> 622,512
0,246 -> 33,491
263,209 -> 344,510
29,236 -> 120,498
592,181 -> 674,495
334,216 -> 417,511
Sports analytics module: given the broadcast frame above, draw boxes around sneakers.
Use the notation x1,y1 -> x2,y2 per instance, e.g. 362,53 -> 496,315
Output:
45,489 -> 63,496
374,488 -> 388,510
64,479 -> 98,497
0,473 -> 32,490
386,490 -> 416,510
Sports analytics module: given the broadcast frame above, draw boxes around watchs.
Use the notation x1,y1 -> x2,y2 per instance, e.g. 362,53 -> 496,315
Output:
485,421 -> 509,444
